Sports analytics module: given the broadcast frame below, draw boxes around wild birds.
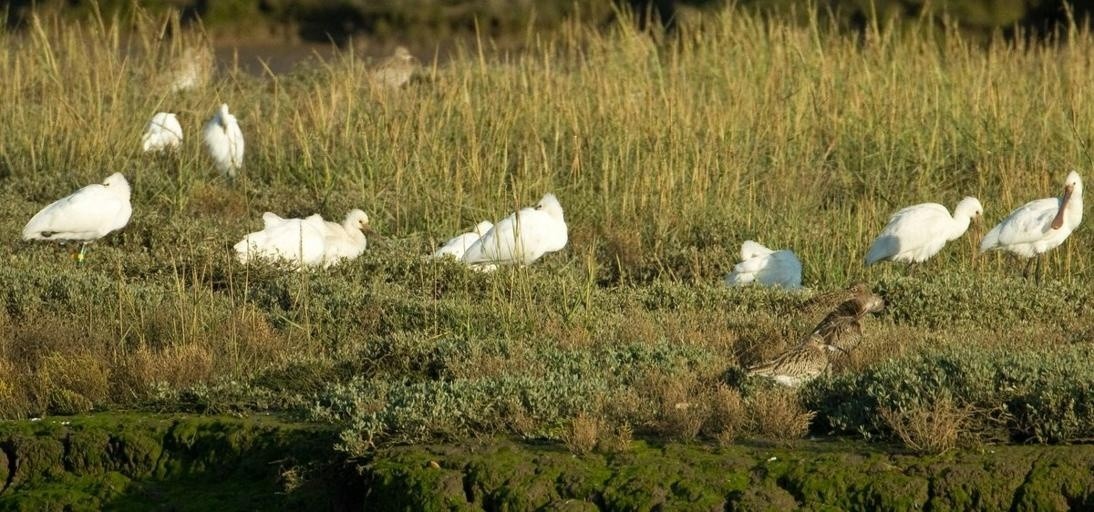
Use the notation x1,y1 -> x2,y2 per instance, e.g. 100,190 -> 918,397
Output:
460,190 -> 572,266
432,219 -> 498,271
724,238 -> 803,293
136,110 -> 186,157
861,195 -> 985,276
198,100 -> 248,187
19,170 -> 135,261
366,44 -> 418,86
744,283 -> 887,403
977,167 -> 1085,285
230,206 -> 372,272
